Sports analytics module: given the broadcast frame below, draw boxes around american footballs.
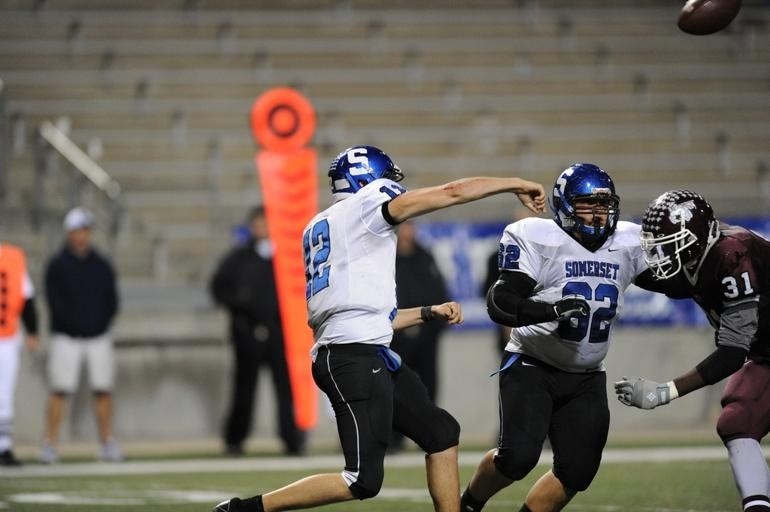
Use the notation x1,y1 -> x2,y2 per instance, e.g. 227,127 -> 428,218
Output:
678,0 -> 742,34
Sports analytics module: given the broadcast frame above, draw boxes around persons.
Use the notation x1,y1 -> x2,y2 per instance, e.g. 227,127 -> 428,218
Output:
614,188 -> 769,512
384,217 -> 451,457
40,204 -> 123,466
458,161 -> 730,511
209,205 -> 310,456
480,248 -> 512,355
212,144 -> 547,511
1,241 -> 42,468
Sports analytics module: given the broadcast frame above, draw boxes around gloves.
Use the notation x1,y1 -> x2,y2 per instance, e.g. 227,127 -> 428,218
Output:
554,294 -> 590,318
614,376 -> 670,409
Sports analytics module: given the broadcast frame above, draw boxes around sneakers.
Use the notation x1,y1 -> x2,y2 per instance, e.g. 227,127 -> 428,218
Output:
1,434 -> 23,464
99,436 -> 121,459
40,445 -> 59,463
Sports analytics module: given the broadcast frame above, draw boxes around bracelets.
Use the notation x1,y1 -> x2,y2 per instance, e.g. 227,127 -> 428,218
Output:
421,304 -> 435,324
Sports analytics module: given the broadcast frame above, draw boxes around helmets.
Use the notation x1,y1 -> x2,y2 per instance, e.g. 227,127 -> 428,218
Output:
328,145 -> 405,195
641,187 -> 721,282
552,163 -> 620,242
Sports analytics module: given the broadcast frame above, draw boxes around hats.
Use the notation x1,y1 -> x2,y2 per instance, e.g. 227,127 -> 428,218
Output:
64,206 -> 96,232
